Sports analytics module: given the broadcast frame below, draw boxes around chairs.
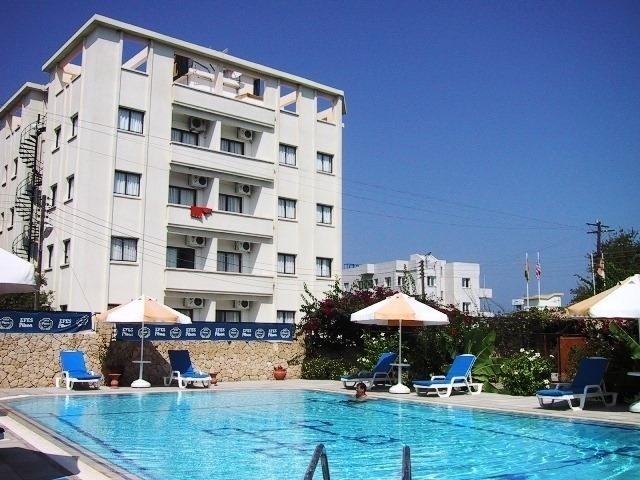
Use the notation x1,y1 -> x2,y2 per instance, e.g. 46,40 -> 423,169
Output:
412,353 -> 485,399
535,356 -> 619,411
55,350 -> 102,390
163,349 -> 213,389
340,352 -> 398,391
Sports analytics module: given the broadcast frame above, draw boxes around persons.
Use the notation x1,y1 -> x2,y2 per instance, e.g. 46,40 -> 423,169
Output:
348,383 -> 368,404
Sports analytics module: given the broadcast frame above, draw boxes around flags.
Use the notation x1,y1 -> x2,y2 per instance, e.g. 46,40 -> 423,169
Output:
597,258 -> 605,280
524,259 -> 530,282
535,260 -> 541,281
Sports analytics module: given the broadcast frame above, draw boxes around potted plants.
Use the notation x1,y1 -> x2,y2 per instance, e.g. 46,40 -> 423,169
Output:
273,365 -> 287,379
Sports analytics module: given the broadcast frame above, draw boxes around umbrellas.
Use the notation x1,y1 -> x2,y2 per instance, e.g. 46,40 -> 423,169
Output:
552,273 -> 640,319
96,295 -> 192,389
349,291 -> 451,394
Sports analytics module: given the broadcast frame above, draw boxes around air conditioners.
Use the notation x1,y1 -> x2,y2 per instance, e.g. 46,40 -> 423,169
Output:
183,298 -> 204,309
235,300 -> 249,310
188,116 -> 208,133
235,183 -> 252,196
237,128 -> 253,141
188,175 -> 208,190
185,235 -> 206,248
235,241 -> 251,252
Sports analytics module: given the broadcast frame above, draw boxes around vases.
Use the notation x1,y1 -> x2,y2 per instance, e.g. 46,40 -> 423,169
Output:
209,374 -> 217,384
108,374 -> 123,388
